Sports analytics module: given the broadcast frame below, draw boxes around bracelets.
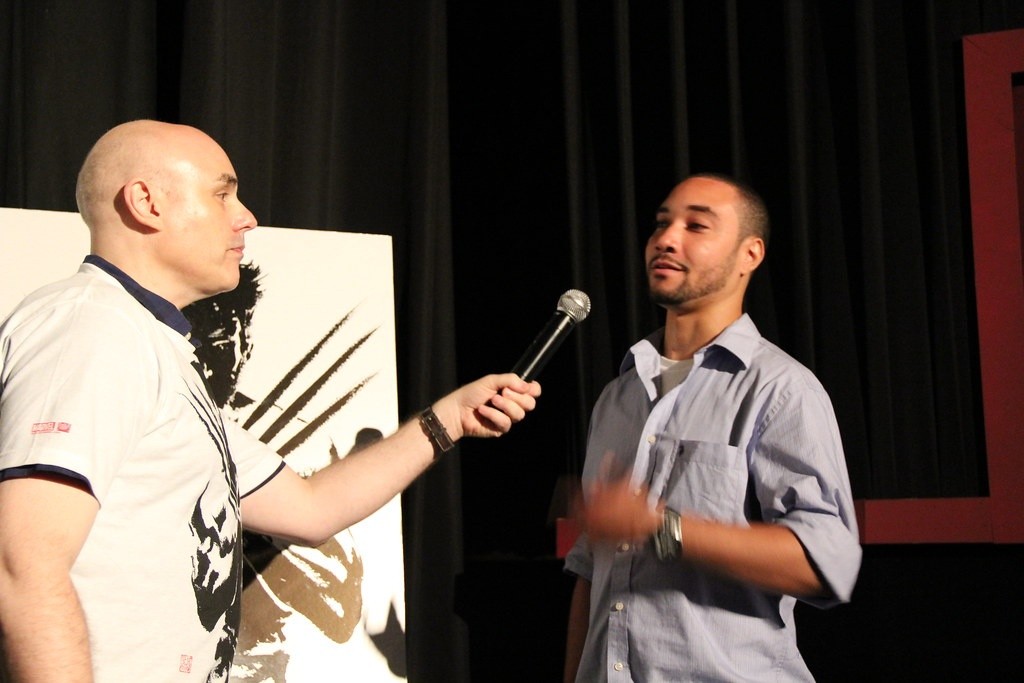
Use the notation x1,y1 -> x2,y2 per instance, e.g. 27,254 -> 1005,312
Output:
654,503 -> 682,561
418,408 -> 455,449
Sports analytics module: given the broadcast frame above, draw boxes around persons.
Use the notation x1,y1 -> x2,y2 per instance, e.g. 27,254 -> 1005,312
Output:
0,119 -> 540,682
546,173 -> 862,683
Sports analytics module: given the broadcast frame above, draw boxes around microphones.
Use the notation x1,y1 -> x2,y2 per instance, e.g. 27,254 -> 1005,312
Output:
485,289 -> 592,412
